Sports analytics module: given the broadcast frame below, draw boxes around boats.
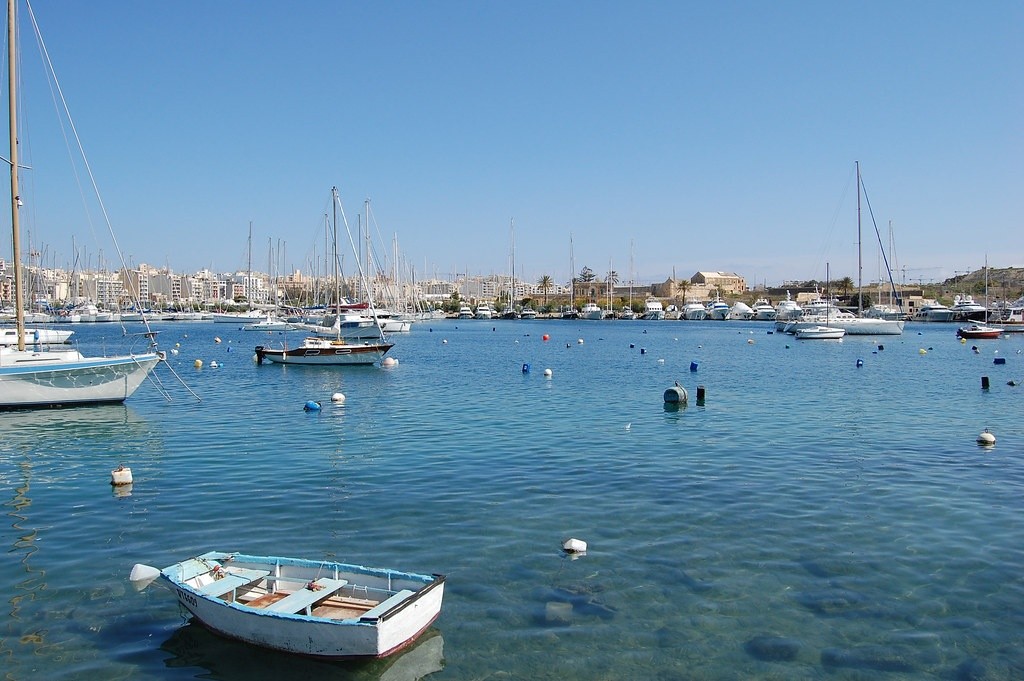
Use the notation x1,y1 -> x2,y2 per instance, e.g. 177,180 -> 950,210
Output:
159,548 -> 447,659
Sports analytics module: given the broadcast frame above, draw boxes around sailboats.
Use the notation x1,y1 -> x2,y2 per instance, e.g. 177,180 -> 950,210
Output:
793,261 -> 846,339
253,183 -> 397,365
952,253 -> 1006,340
0,161 -> 1024,346
0,0 -> 168,407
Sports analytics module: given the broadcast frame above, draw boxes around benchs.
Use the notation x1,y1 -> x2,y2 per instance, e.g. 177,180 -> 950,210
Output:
264,577 -> 348,614
198,570 -> 270,598
359,589 -> 417,623
